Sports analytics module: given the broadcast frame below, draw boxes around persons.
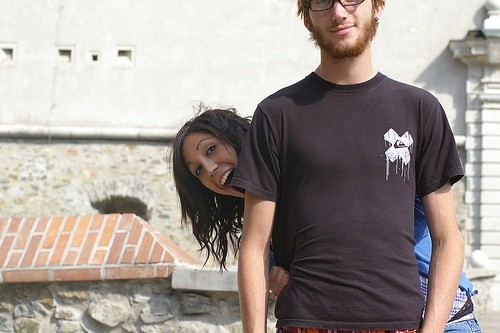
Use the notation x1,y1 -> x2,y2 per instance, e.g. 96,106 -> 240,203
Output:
228,0 -> 465,333
170,106 -> 482,333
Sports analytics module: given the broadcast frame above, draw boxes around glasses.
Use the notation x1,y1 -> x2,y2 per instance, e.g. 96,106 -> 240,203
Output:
304,0 -> 364,12
